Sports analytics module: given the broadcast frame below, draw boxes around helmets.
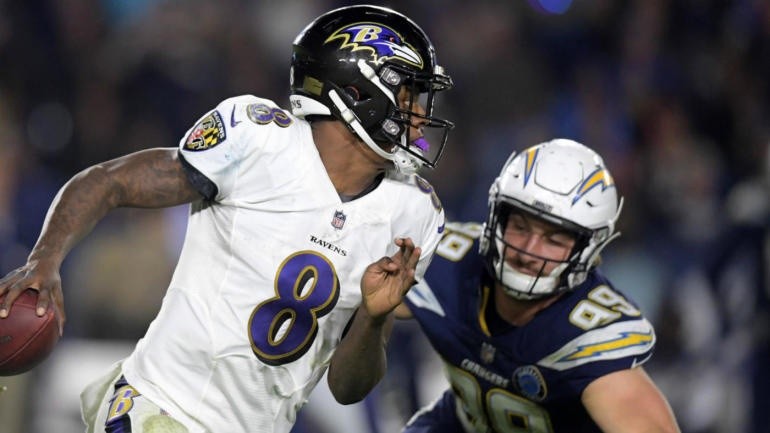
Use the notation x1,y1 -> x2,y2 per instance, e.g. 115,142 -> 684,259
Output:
479,138 -> 625,302
289,4 -> 454,173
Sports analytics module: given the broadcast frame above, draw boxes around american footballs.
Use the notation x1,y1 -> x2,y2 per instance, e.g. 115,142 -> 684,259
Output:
0,287 -> 60,377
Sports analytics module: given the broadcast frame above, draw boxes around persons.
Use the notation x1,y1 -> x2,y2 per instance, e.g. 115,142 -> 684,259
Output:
0,5 -> 456,433
392,139 -> 682,433
0,0 -> 770,433
